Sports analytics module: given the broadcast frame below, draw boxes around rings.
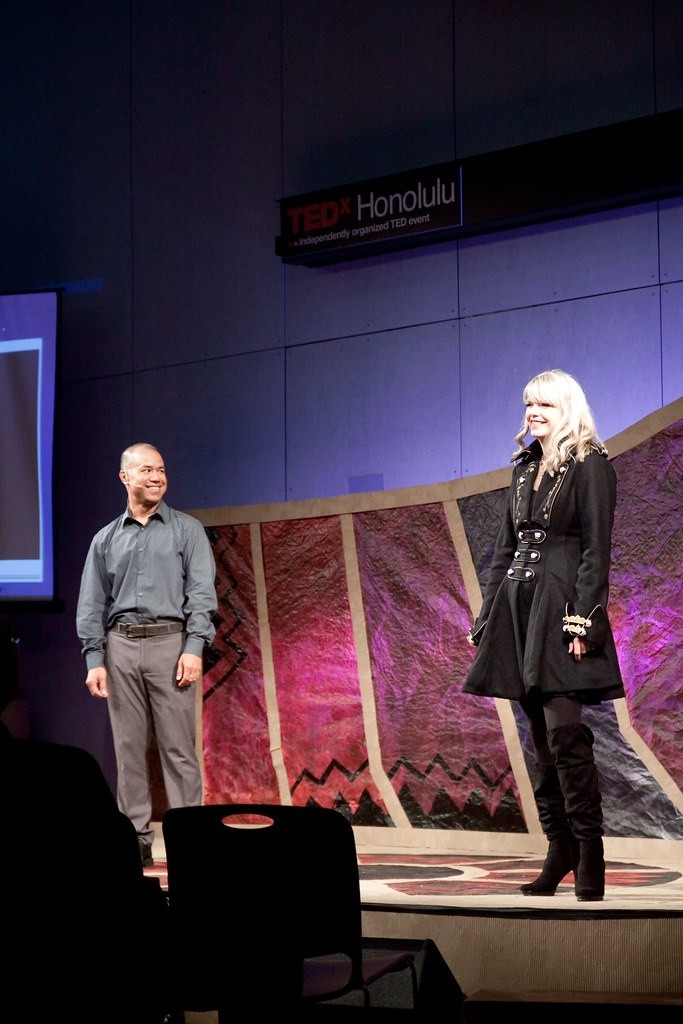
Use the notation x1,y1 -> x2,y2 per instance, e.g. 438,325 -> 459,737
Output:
190,676 -> 194,679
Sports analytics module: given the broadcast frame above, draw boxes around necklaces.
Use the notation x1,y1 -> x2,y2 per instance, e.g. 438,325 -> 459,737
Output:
540,456 -> 547,476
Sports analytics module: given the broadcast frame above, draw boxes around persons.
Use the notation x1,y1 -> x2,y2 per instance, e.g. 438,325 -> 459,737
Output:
0,613 -> 168,1024
463,370 -> 624,903
76,442 -> 217,867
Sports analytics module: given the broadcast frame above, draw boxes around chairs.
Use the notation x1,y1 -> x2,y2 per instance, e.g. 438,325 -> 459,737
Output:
161,803 -> 417,1024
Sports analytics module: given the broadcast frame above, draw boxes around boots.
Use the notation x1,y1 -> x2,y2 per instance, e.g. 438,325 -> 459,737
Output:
520,726 -> 577,896
547,722 -> 606,902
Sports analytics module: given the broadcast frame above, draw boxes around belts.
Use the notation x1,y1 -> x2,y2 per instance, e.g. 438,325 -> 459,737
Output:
111,621 -> 186,639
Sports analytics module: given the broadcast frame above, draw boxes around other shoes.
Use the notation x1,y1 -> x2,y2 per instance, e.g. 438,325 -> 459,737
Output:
141,844 -> 154,867
